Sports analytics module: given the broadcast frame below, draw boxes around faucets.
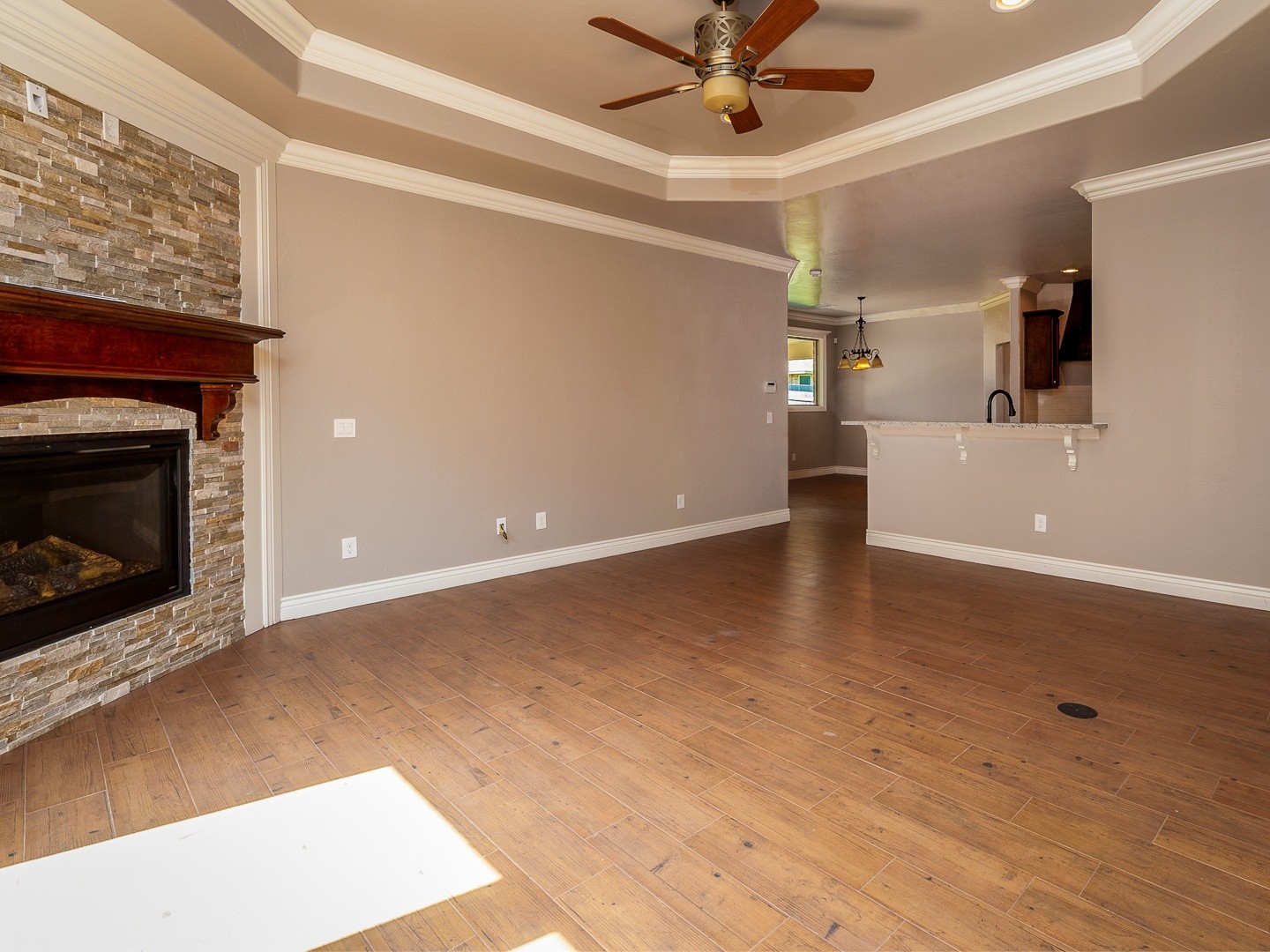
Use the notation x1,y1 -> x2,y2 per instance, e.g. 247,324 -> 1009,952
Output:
986,390 -> 1017,424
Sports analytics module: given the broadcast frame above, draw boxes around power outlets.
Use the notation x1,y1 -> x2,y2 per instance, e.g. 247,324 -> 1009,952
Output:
677,495 -> 685,509
792,454 -> 796,461
496,517 -> 507,536
1034,514 -> 1048,533
342,538 -> 358,559
536,512 -> 547,530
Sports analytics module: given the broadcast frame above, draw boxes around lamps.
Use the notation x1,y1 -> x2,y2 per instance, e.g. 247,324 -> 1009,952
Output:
837,297 -> 883,371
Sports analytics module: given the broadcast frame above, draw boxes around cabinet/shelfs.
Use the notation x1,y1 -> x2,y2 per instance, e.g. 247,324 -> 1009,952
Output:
1021,309 -> 1065,388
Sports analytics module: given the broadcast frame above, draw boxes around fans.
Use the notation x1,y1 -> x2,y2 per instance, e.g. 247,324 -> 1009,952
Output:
590,1 -> 875,135
